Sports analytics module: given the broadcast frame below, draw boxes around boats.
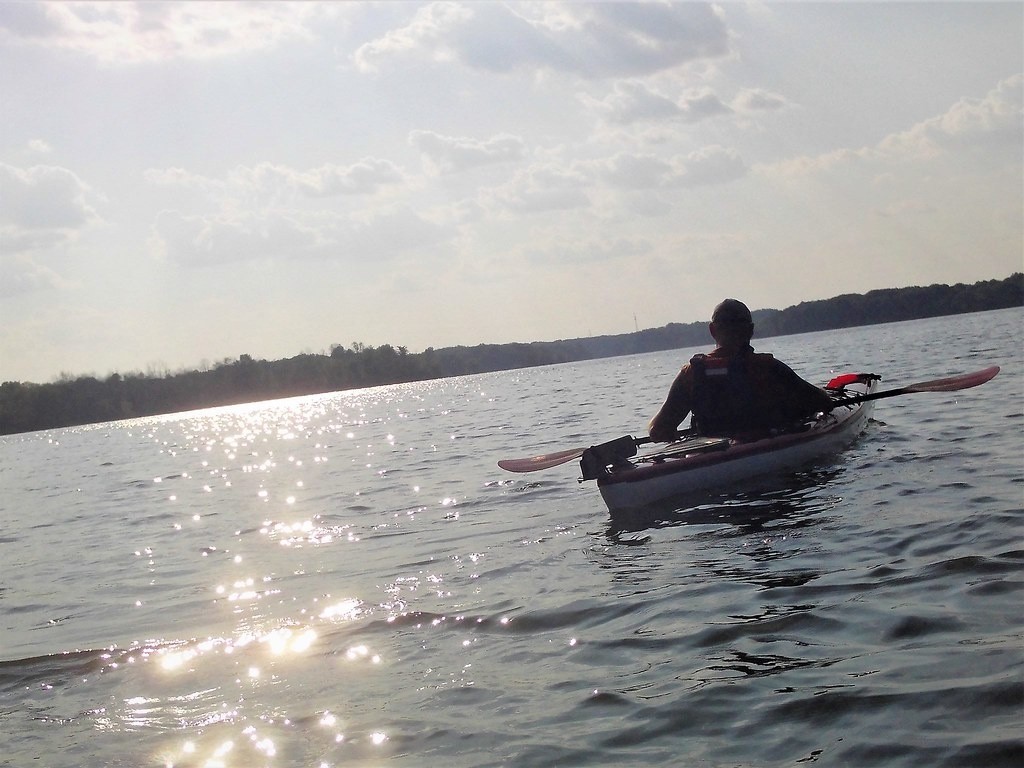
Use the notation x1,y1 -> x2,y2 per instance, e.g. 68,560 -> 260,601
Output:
576,368 -> 883,516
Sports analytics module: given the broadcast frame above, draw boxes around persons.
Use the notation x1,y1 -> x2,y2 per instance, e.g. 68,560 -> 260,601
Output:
649,298 -> 833,442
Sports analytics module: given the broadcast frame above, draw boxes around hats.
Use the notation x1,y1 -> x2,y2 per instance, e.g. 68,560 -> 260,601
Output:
713,299 -> 752,323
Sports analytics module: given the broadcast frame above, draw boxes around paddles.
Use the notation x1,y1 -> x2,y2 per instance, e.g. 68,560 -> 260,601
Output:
498,366 -> 1001,472
